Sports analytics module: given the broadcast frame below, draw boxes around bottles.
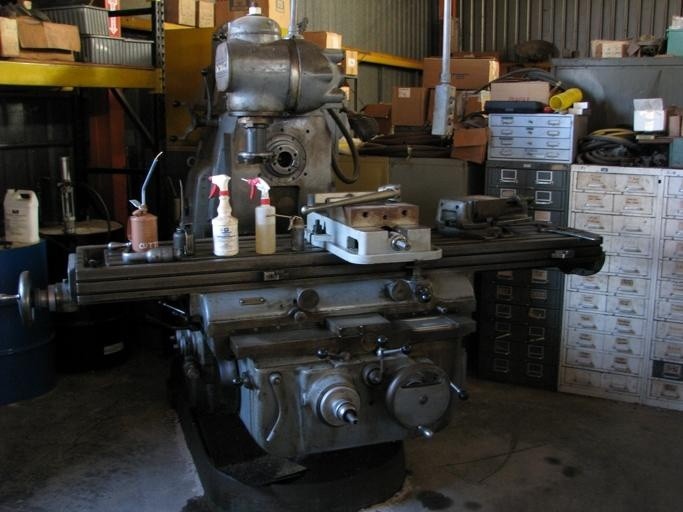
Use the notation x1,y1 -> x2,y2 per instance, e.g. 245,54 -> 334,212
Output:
173,223 -> 195,257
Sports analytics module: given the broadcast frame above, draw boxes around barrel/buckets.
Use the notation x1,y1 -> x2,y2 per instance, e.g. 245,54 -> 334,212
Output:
4,188 -> 39,243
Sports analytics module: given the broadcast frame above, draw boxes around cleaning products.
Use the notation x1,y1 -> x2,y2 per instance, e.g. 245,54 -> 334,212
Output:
205,175 -> 239,259
239,178 -> 277,254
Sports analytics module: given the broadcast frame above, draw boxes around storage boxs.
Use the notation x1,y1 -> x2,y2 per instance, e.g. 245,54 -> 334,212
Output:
359,57 -> 499,165
